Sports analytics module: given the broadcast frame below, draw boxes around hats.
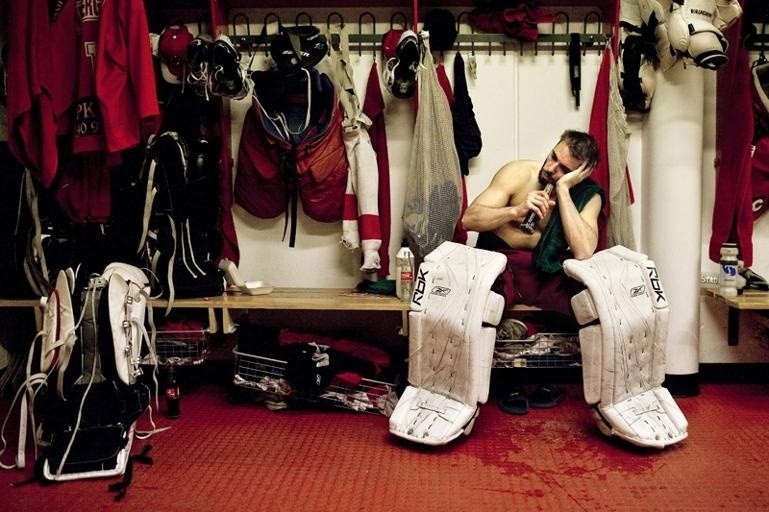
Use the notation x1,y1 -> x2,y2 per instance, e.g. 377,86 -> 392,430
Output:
158,25 -> 194,84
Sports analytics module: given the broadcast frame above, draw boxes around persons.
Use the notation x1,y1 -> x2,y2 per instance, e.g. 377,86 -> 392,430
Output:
387,128 -> 689,449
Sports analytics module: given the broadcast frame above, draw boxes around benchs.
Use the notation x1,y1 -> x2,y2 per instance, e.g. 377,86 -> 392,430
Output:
1,287 -> 545,342
701,279 -> 769,346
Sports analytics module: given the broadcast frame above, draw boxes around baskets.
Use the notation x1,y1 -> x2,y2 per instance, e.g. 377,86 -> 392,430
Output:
491,333 -> 582,369
232,344 -> 399,415
140,329 -> 209,366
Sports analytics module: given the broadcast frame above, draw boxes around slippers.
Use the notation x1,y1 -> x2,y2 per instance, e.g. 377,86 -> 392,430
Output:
528,384 -> 566,409
497,391 -> 529,415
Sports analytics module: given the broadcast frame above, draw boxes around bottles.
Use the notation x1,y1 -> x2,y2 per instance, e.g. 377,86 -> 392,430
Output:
401,250 -> 414,304
165,365 -> 181,418
719,243 -> 739,287
521,183 -> 554,235
396,239 -> 415,299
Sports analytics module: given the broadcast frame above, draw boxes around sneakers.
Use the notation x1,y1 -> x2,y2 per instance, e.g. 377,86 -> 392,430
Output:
186,33 -> 213,101
205,34 -> 249,101
390,29 -> 419,98
